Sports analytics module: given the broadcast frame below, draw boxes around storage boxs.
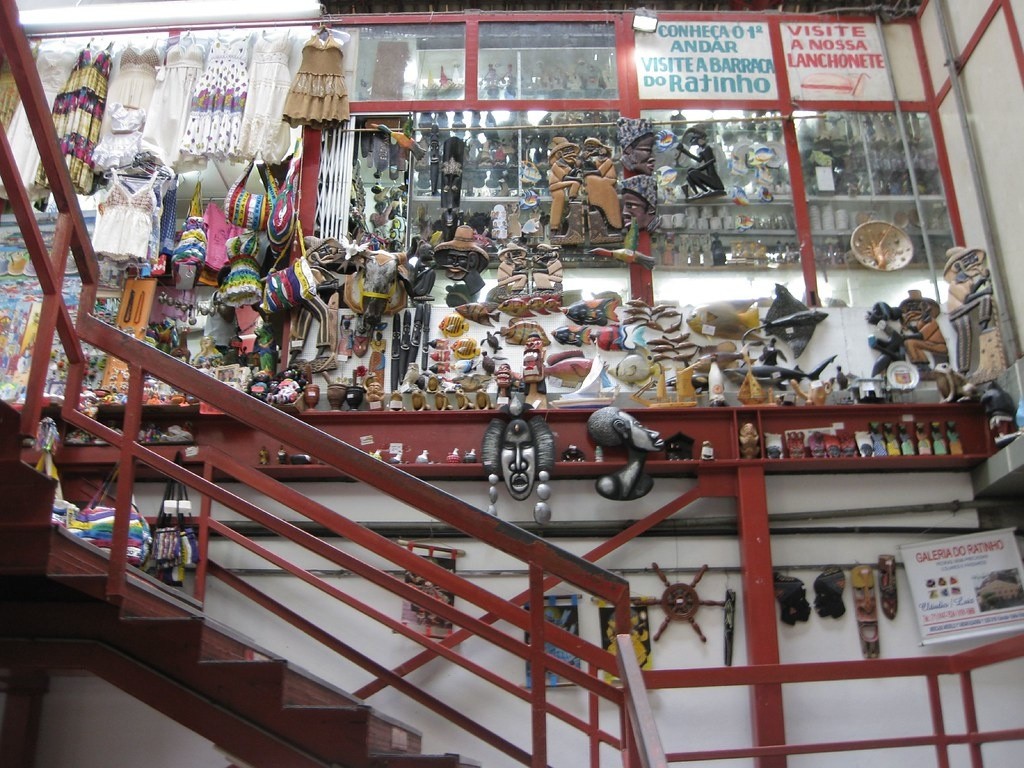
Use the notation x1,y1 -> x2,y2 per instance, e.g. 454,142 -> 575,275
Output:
273,392 -> 305,414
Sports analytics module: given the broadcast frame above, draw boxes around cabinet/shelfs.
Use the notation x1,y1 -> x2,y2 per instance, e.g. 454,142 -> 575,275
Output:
409,196 -> 959,270
21,405 -> 1015,481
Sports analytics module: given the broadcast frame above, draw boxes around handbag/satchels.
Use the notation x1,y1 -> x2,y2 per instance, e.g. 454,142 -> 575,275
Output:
264,221 -> 318,311
172,181 -> 208,266
143,451 -> 202,589
71,458 -> 153,566
33,452 -> 79,528
267,137 -> 302,259
223,157 -> 276,231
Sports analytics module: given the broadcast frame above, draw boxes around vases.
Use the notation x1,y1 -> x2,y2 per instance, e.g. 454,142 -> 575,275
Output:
327,384 -> 348,410
304,384 -> 319,412
346,387 -> 366,410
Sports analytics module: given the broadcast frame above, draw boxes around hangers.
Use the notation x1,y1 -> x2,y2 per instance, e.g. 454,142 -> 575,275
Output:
103,151 -> 179,181
124,97 -> 139,110
176,21 -> 330,44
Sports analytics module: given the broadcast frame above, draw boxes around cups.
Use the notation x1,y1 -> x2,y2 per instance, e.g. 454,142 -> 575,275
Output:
658,205 -> 734,229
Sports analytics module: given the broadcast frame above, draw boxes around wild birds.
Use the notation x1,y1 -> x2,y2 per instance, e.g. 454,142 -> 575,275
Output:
587,217 -> 656,271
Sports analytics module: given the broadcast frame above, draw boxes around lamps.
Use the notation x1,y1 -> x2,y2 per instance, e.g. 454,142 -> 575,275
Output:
631,7 -> 658,32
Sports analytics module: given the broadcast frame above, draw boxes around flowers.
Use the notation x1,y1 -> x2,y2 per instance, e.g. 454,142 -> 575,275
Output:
336,366 -> 367,387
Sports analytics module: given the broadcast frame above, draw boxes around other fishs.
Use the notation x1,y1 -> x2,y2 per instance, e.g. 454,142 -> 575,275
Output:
519,160 -> 543,210
654,129 -> 679,187
427,283 -> 838,392
